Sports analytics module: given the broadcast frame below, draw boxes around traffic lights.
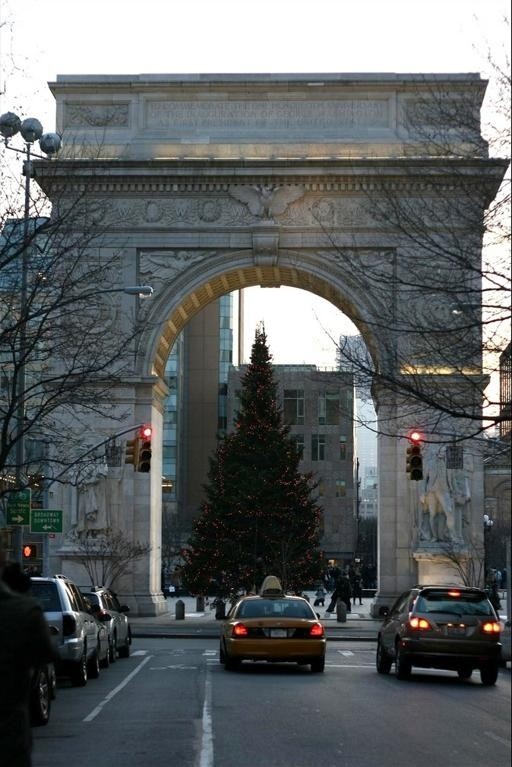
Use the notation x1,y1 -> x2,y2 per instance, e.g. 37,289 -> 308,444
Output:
124,438 -> 136,465
410,445 -> 425,481
410,432 -> 420,442
136,439 -> 151,473
22,544 -> 36,559
406,446 -> 413,472
143,427 -> 152,437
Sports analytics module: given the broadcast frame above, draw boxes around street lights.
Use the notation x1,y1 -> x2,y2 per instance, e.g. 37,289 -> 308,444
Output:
448,301 -> 512,315
484,514 -> 495,589
0,109 -> 61,575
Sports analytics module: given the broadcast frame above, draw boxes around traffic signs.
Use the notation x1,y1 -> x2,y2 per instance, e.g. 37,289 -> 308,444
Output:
28,509 -> 63,535
5,488 -> 31,526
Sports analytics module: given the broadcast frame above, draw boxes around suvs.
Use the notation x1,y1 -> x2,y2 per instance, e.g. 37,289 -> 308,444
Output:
375,585 -> 504,688
77,585 -> 132,663
27,574 -> 102,688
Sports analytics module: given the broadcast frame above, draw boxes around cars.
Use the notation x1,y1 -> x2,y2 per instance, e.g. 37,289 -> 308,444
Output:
30,659 -> 57,728
80,596 -> 112,679
216,575 -> 328,674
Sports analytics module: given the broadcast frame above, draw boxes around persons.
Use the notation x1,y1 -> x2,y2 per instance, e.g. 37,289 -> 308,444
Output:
490,585 -> 504,621
419,441 -> 457,542
161,585 -> 167,600
314,575 -> 363,613
29,565 -> 42,576
72,444 -> 111,541
169,584 -> 175,597
296,592 -> 302,596
0,559 -> 57,767
